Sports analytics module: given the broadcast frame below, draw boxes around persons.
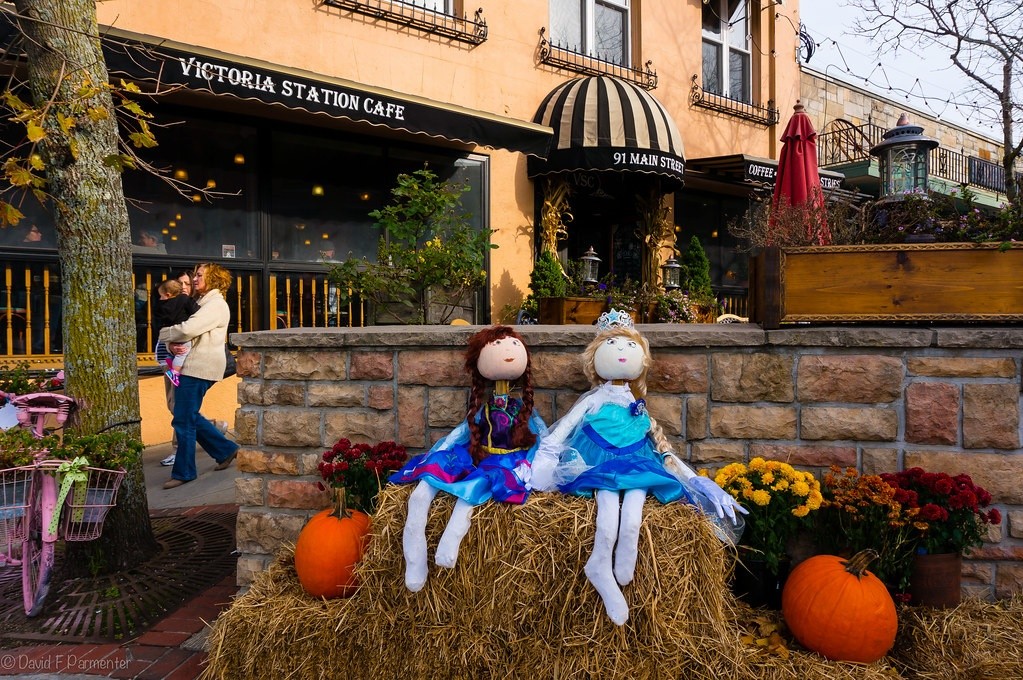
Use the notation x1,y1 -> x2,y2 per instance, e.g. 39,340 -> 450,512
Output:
162,263 -> 243,489
531,325 -> 748,625
159,267 -> 227,467
400,325 -> 551,593
155,277 -> 203,386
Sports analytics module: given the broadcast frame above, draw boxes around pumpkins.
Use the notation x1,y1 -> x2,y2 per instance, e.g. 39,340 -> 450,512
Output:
294,486 -> 371,600
781,549 -> 899,662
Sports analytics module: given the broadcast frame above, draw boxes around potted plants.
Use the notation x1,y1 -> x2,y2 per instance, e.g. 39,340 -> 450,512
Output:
0,426 -> 58,520
52,428 -> 146,523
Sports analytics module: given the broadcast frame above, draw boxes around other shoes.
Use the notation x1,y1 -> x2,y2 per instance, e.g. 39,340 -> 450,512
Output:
215,447 -> 239,470
165,478 -> 183,488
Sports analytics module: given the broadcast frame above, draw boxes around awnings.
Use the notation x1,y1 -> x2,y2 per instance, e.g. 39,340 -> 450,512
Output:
1,3 -> 554,162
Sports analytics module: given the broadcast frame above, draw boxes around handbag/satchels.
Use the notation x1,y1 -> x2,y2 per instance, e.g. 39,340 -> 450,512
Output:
207,343 -> 238,388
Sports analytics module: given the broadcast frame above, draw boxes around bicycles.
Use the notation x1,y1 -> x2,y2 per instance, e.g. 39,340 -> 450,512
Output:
1,371 -> 126,620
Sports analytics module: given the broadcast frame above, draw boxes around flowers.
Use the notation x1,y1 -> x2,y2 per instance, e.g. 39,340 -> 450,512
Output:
577,267 -> 728,325
878,467 -> 1002,556
820,465 -> 928,577
317,438 -> 407,514
697,456 -> 822,577
892,183 -> 1023,253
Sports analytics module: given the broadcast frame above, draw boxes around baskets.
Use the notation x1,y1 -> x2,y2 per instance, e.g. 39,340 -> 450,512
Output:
56,454 -> 125,541
0,447 -> 35,545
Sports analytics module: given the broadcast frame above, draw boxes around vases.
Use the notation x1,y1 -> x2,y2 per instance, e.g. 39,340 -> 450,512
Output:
912,552 -> 961,610
731,555 -> 794,611
830,544 -> 855,560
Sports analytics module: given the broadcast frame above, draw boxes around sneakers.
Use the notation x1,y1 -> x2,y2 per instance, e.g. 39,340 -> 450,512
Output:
161,454 -> 177,465
165,369 -> 180,388
